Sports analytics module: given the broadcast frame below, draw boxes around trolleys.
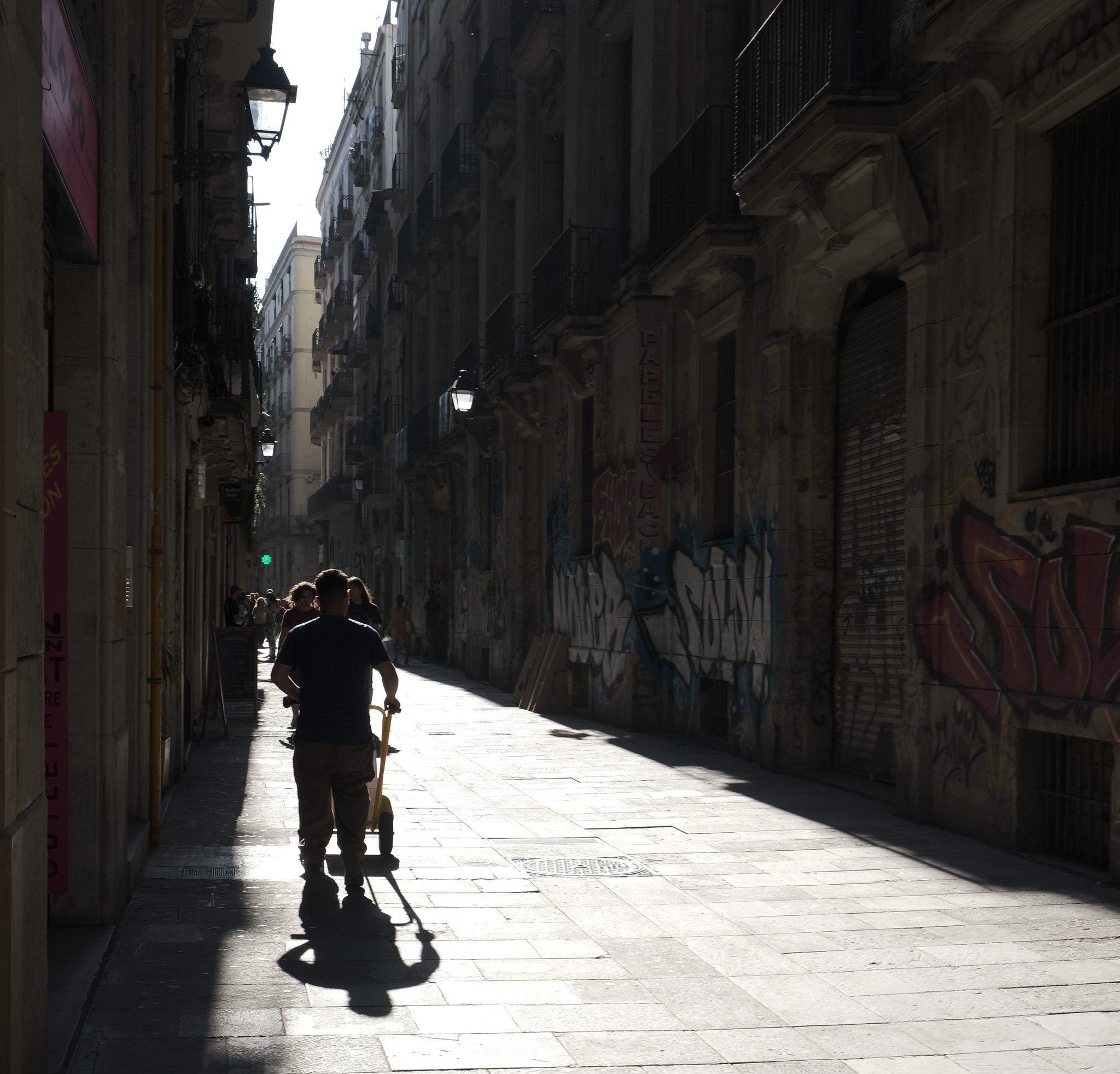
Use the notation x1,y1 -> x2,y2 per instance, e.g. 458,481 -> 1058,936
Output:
299,703 -> 395,857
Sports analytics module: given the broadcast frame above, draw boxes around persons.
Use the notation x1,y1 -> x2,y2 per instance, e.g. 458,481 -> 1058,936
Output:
224,585 -> 321,659
277,581 -> 321,726
346,576 -> 383,705
384,594 -> 411,665
271,568 -> 401,885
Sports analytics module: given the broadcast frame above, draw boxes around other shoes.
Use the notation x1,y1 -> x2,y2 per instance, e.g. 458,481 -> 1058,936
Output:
423,659 -> 435,664
304,885 -> 325,904
291,715 -> 299,727
404,660 -> 409,665
270,654 -> 276,659
345,859 -> 365,888
393,658 -> 398,664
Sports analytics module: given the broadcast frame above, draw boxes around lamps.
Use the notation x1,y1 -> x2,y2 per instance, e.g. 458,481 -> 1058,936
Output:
447,370 -> 500,437
173,47 -> 297,183
254,428 -> 277,466
353,473 -> 364,496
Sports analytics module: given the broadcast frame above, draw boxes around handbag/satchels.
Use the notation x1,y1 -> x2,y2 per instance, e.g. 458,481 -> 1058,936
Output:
381,634 -> 396,661
405,607 -> 416,635
